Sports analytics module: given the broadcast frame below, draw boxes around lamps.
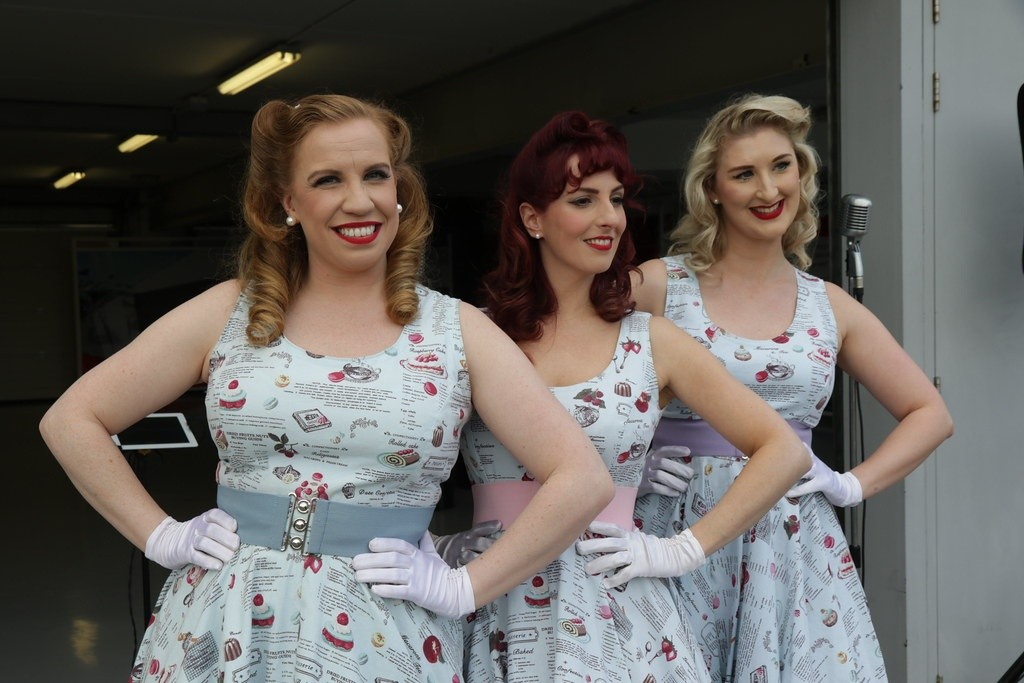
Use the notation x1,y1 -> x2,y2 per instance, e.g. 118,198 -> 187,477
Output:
218,46 -> 301,97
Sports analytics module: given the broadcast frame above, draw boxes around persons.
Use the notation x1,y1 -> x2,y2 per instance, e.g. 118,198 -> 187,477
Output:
427,112 -> 814,683
38,94 -> 617,683
618,95 -> 954,683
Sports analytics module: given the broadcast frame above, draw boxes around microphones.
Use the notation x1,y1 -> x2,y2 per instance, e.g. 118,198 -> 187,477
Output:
837,194 -> 873,304
